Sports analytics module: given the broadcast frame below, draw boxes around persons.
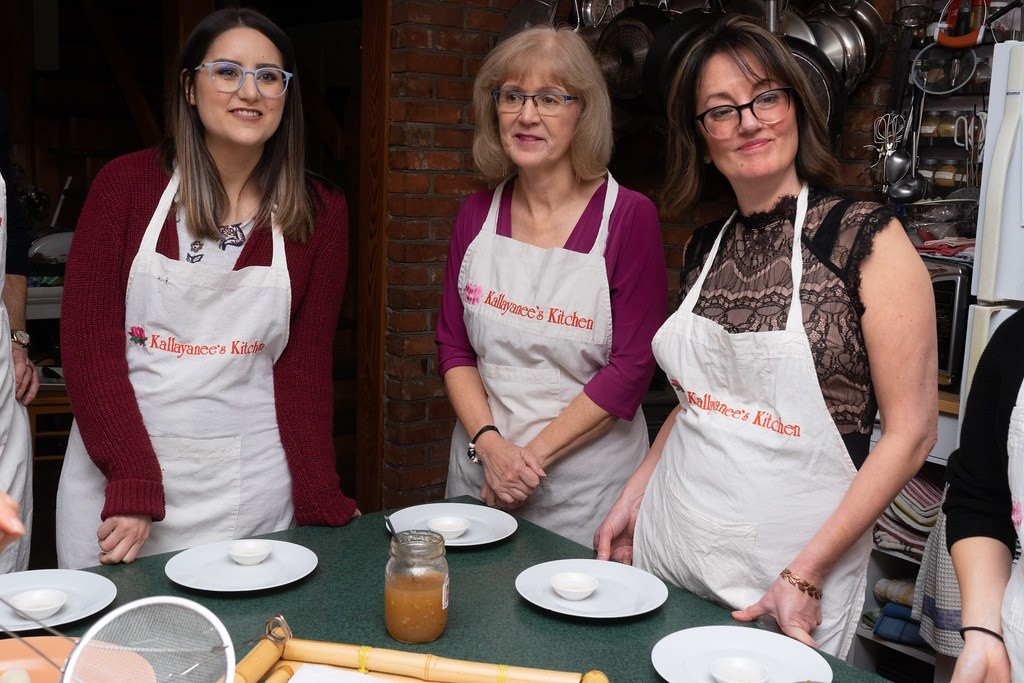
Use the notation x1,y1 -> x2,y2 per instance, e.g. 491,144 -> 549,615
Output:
55,9 -> 362,567
592,20 -> 939,659
435,23 -> 665,548
941,309 -> 1024,683
0,173 -> 40,575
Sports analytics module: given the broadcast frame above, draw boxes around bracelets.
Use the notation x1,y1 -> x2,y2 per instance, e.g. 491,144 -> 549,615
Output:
780,568 -> 823,600
467,425 -> 501,464
960,627 -> 1005,644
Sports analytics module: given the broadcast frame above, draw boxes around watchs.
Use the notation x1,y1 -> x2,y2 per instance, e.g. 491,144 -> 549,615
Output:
11,329 -> 30,348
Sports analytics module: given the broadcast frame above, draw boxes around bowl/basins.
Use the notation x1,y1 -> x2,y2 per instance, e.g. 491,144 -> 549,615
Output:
226,542 -> 272,565
10,589 -> 67,619
552,572 -> 598,600
707,656 -> 770,682
427,516 -> 470,539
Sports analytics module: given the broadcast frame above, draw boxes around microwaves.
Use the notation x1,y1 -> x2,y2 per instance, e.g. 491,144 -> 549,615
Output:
922,258 -> 972,393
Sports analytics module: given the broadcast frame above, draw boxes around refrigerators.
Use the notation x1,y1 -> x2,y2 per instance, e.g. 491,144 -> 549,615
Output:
954,39 -> 1024,454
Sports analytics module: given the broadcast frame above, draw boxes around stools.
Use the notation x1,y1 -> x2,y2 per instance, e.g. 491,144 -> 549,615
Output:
27,356 -> 77,476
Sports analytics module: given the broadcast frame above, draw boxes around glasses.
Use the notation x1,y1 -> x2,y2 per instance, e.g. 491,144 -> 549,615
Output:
194,62 -> 292,98
695,86 -> 794,140
492,91 -> 579,115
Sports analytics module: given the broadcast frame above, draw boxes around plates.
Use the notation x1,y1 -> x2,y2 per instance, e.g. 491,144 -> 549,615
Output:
0,637 -> 156,683
386,503 -> 518,546
1,569 -> 117,631
165,538 -> 318,591
515,559 -> 669,618
651,625 -> 834,683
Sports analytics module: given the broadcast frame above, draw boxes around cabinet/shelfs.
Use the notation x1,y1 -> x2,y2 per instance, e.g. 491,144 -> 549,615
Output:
844,390 -> 962,683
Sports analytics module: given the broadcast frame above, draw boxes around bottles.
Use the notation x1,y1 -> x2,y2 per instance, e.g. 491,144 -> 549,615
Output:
920,1 -> 1008,192
383,531 -> 451,643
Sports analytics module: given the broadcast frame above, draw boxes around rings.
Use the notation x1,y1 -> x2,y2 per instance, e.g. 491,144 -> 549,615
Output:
27,365 -> 33,374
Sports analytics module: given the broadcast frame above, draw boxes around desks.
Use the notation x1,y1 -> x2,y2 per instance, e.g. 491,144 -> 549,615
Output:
0,494 -> 893,683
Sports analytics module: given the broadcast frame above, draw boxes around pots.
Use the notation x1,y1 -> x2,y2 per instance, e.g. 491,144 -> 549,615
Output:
501,1 -> 886,160
